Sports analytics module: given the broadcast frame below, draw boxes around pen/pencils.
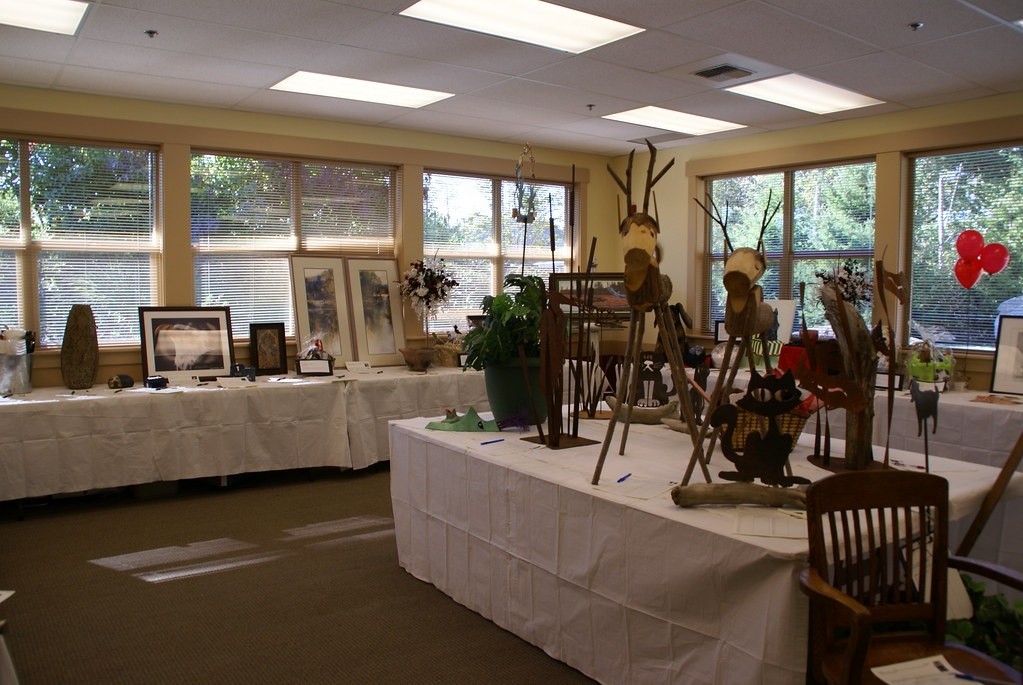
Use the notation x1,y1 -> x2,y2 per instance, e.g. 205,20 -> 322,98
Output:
890,459 -> 924,469
197,383 -> 209,386
277,376 -> 286,381
481,439 -> 504,445
954,673 -> 1013,685
3,393 -> 14,398
617,473 -> 631,482
115,389 -> 122,393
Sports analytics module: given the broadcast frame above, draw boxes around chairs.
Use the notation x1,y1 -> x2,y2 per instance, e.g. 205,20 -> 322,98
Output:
796,470 -> 1023,685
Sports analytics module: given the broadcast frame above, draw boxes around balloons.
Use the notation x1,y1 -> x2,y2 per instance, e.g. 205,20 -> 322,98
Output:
954,230 -> 1010,289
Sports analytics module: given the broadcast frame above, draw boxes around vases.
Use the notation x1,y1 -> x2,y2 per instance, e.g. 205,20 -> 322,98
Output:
398,348 -> 438,371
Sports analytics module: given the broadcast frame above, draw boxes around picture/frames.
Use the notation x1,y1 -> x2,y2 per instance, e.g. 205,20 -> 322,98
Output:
289,254 -> 355,369
550,272 -> 640,322
138,305 -> 234,382
991,314 -> 1023,394
714,319 -> 741,344
249,322 -> 289,378
350,257 -> 406,369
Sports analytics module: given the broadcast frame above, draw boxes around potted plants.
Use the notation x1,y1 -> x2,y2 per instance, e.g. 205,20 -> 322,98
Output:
466,272 -> 563,424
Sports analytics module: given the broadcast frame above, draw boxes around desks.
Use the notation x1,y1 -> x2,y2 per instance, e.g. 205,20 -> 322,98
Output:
386,417 -> 1023,685
332,359 -> 605,477
0,379 -> 350,521
619,360 -> 1023,573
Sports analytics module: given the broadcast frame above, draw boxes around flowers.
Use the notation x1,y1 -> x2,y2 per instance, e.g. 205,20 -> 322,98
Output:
396,258 -> 460,350
